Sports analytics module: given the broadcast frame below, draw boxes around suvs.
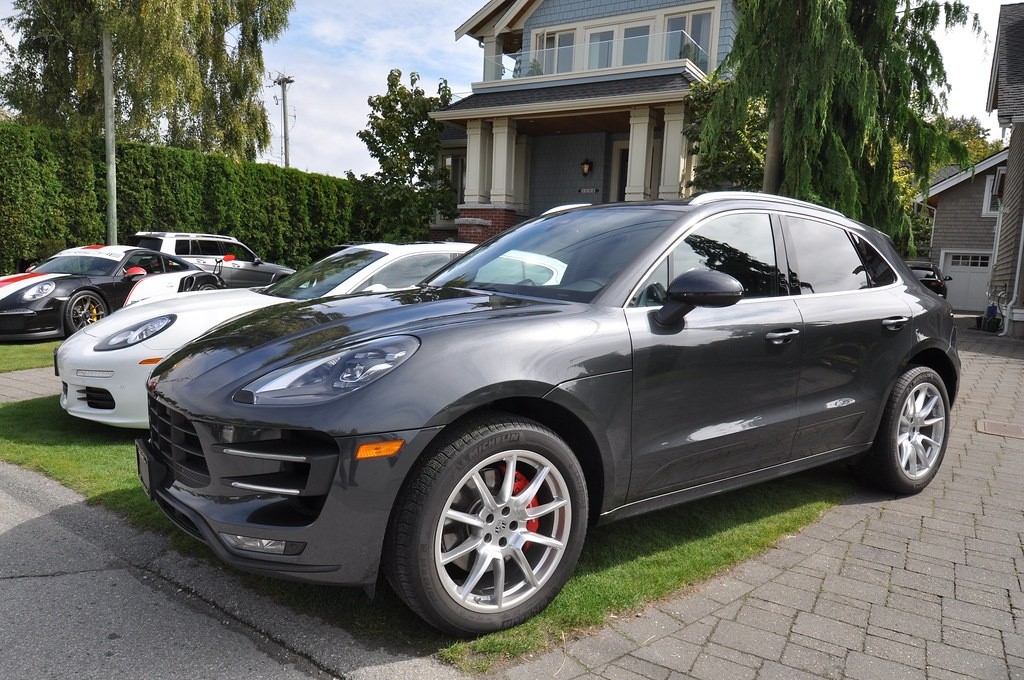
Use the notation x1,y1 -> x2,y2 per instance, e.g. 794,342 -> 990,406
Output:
133,229 -> 317,291
903,256 -> 953,299
133,188 -> 964,641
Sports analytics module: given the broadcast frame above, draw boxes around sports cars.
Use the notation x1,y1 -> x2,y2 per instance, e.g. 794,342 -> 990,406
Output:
0,244 -> 229,342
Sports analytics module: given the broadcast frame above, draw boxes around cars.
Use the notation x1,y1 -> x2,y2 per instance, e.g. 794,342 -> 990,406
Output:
55,241 -> 604,432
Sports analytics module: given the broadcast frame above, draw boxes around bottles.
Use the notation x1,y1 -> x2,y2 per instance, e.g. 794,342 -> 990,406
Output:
986,302 -> 997,318
